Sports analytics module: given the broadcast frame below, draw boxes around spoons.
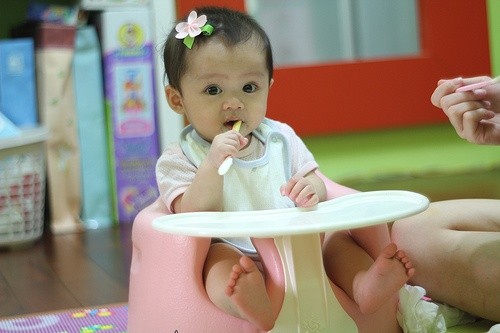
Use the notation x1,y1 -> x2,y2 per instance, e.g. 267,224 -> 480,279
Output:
218,120 -> 243,175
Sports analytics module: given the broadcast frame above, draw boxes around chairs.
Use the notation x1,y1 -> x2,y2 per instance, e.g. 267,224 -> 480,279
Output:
128,165 -> 432,333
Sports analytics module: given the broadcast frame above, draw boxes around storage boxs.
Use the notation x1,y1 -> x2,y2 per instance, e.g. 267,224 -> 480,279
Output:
1,5 -> 162,246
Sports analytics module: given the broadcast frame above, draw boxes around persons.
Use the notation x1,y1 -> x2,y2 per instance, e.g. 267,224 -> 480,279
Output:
390,74 -> 500,323
154,6 -> 416,331
